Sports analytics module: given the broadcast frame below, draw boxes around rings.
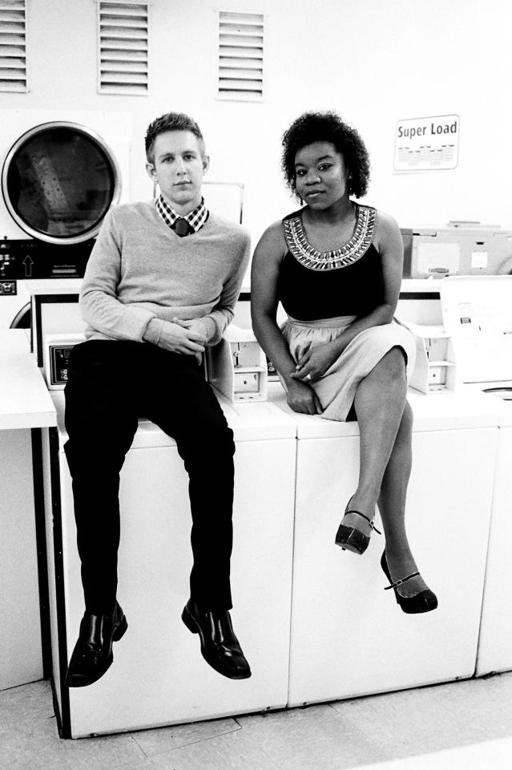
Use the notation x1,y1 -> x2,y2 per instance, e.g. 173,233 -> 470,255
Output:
307,374 -> 312,381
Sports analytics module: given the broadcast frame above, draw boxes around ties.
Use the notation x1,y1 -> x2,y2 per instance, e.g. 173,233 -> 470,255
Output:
174,217 -> 190,239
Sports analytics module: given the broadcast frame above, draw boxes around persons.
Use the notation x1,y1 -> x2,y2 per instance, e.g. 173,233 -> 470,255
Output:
64,113 -> 252,687
251,113 -> 437,615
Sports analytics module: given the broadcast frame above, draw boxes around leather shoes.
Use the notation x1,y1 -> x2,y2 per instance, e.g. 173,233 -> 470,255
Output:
66,601 -> 128,687
180,597 -> 255,680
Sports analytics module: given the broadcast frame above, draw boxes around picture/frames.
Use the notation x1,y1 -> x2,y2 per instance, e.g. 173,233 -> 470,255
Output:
45,337 -> 85,391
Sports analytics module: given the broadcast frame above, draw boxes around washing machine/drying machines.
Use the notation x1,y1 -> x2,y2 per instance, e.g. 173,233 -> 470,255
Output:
26,269 -> 512,739
2,80 -> 511,270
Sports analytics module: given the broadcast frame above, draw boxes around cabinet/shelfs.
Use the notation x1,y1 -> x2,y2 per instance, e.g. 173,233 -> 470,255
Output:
208,332 -> 269,403
409,326 -> 459,396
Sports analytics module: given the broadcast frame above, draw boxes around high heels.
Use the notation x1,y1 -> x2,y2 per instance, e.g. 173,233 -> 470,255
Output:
380,548 -> 439,614
336,494 -> 378,554
